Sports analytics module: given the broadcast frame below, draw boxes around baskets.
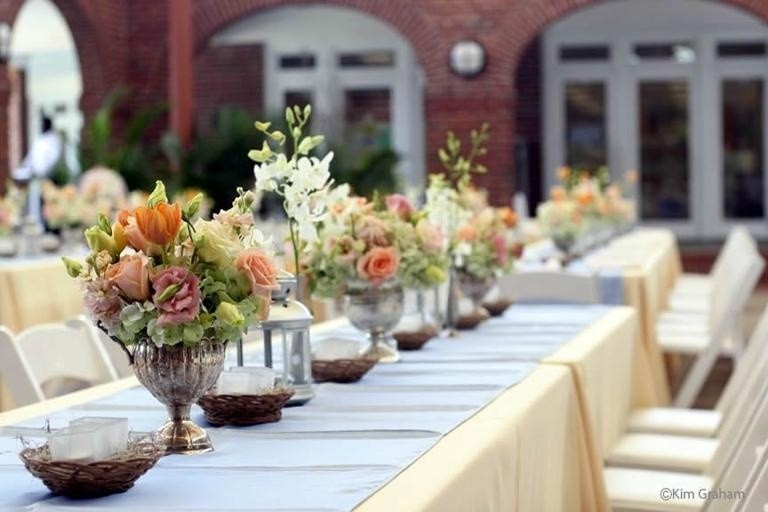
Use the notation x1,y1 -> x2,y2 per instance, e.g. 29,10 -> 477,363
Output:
197,393 -> 294,426
485,299 -> 514,316
20,431 -> 165,501
394,327 -> 436,350
313,356 -> 381,383
454,306 -> 490,329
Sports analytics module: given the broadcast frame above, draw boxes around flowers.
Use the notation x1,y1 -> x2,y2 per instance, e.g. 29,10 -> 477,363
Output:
536,161 -> 649,219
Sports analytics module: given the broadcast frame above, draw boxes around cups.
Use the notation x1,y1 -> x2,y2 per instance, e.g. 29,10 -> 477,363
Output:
46,414 -> 129,465
215,363 -> 276,398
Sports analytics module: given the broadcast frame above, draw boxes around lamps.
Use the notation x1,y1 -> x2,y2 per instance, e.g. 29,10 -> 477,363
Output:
446,38 -> 487,79
